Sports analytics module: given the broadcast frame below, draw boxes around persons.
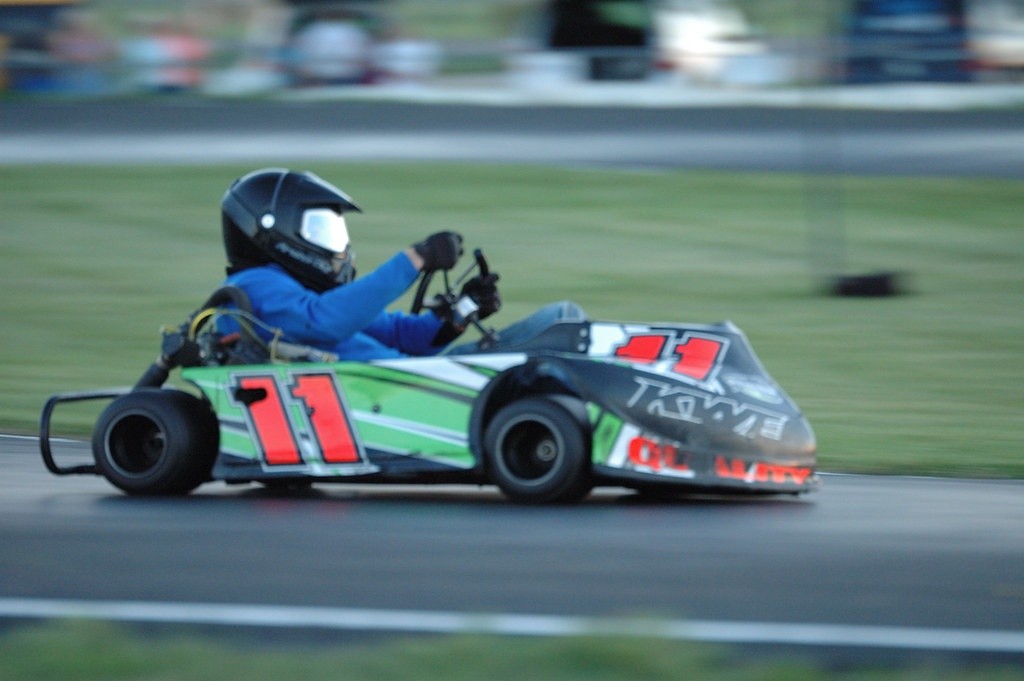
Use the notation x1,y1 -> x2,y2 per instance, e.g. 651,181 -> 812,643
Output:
214,168 -> 503,362
45,10 -> 211,95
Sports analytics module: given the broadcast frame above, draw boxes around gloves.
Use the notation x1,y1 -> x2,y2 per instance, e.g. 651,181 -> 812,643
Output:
413,229 -> 466,273
461,273 -> 503,320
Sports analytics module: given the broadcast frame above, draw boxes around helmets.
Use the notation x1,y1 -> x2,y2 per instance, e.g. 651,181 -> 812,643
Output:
219,165 -> 360,294
